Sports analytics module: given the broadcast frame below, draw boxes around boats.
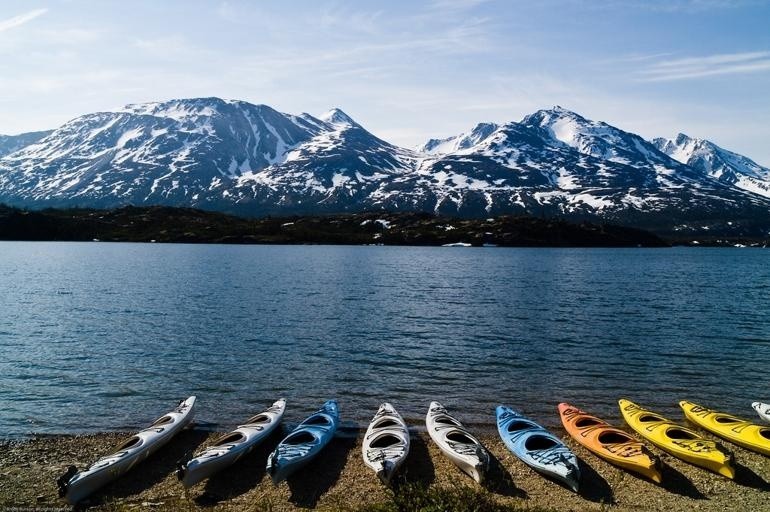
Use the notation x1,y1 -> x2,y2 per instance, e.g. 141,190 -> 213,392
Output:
425,400 -> 490,486
558,402 -> 661,484
361,402 -> 410,485
680,400 -> 770,456
266,400 -> 339,474
496,405 -> 581,494
174,398 -> 286,490
57,396 -> 197,504
618,398 -> 736,479
